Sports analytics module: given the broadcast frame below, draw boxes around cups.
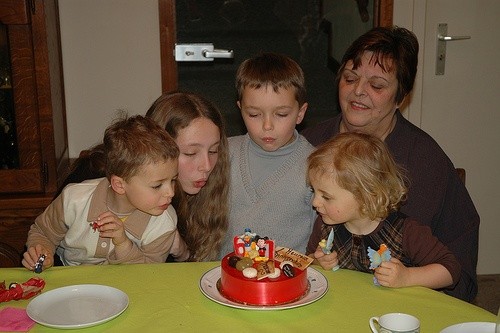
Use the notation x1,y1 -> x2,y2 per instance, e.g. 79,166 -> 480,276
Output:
369,312 -> 421,333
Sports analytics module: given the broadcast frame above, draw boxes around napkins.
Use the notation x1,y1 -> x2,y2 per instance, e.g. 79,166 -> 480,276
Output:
0,307 -> 36,332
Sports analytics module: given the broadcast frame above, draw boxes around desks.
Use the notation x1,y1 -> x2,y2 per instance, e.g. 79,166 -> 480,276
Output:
0,260 -> 500,333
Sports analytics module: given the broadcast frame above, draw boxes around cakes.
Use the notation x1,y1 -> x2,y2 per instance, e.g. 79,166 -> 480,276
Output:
220,229 -> 314,306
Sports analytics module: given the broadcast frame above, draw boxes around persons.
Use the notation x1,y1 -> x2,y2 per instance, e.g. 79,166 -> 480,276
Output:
51,91 -> 231,266
216,53 -> 319,260
307,132 -> 460,289
300,27 -> 481,301
20,116 -> 180,271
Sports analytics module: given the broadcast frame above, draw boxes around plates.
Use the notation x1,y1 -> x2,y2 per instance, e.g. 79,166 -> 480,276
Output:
198,264 -> 329,310
25,284 -> 130,330
439,322 -> 496,333
